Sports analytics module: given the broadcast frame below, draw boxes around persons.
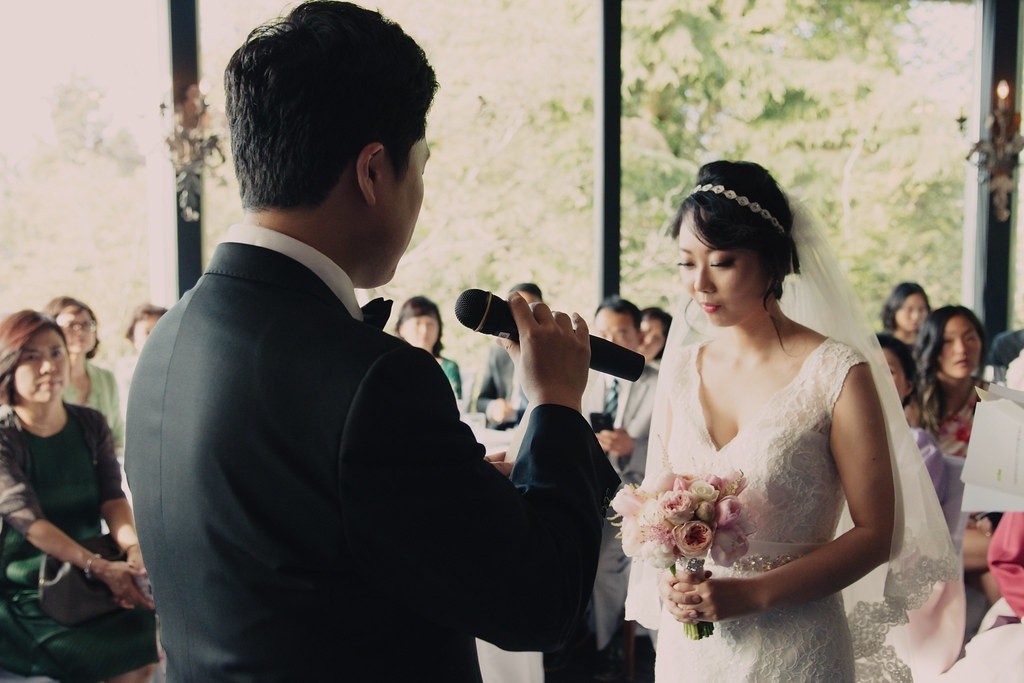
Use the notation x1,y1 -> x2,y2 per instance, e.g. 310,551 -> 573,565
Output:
0,310 -> 162,682
580,297 -> 672,651
127,304 -> 167,349
127,1 -> 619,683
872,281 -> 1023,682
477,284 -> 544,429
43,296 -> 125,459
625,161 -> 960,683
394,296 -> 462,399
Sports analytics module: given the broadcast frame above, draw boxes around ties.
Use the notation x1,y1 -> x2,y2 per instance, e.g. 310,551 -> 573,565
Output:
604,380 -> 619,419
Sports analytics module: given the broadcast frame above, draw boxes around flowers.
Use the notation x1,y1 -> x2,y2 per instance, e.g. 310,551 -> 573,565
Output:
608,433 -> 749,641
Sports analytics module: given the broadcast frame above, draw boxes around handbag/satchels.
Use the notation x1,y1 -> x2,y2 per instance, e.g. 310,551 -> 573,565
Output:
40,533 -> 148,630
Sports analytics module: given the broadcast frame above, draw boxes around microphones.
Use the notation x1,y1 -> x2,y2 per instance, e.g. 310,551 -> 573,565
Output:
455,289 -> 645,382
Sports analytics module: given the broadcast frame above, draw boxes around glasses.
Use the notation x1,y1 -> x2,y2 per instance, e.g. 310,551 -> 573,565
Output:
58,319 -> 97,334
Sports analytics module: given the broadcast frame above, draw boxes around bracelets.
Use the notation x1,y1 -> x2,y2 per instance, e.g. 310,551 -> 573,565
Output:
84,553 -> 103,578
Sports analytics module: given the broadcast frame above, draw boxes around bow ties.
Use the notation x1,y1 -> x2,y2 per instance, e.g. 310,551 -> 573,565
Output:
359,297 -> 392,333
